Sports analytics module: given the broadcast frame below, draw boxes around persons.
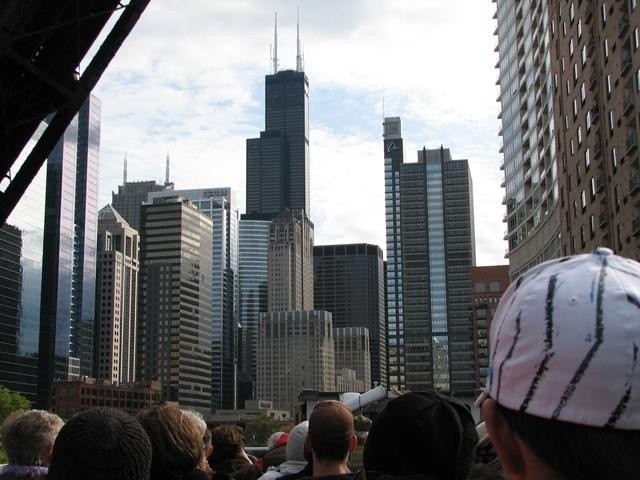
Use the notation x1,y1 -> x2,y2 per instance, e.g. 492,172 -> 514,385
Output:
0,391 -> 501,480
476,248 -> 640,480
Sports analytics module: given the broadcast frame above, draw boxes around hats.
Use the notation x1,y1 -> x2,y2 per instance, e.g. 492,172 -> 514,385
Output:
48,407 -> 152,480
365,388 -> 479,480
473,247 -> 640,426
311,400 -> 354,437
475,421 -> 489,447
